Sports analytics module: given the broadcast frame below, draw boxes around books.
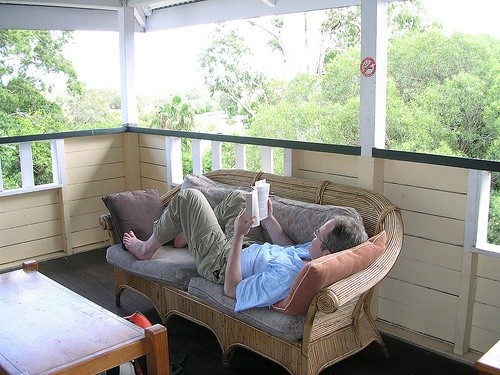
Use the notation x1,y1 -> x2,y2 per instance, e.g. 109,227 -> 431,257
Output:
245,178 -> 270,228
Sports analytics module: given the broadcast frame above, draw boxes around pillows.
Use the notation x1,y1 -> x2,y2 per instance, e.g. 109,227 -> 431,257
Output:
101,188 -> 165,251
271,230 -> 387,314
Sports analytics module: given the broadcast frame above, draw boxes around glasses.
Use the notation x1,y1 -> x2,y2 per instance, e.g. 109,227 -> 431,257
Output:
313,229 -> 335,254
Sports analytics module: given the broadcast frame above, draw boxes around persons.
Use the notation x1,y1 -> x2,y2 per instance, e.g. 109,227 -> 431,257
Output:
123,188 -> 368,313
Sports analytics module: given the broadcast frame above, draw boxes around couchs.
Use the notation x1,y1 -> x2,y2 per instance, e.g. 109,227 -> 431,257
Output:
99,169 -> 404,375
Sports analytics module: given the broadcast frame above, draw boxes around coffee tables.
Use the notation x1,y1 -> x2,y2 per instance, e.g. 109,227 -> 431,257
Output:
0,260 -> 169,375
